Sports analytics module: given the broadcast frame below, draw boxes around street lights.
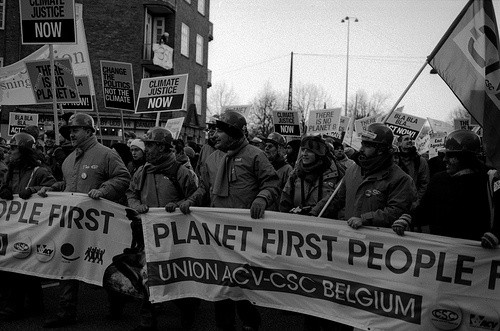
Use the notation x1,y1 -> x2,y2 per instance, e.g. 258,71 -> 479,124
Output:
341,16 -> 359,117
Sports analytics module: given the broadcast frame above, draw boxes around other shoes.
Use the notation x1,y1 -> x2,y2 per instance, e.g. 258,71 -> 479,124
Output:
0,304 -> 43,319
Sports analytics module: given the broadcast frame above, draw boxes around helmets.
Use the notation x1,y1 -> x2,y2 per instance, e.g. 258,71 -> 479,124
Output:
4,133 -> 36,151
261,132 -> 286,146
140,128 -> 172,147
65,114 -> 96,133
443,130 -> 482,154
361,123 -> 394,147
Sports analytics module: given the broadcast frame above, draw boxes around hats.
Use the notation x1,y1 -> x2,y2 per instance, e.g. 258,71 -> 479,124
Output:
130,139 -> 145,151
204,116 -> 220,124
249,136 -> 266,145
300,136 -> 326,155
217,110 -> 247,131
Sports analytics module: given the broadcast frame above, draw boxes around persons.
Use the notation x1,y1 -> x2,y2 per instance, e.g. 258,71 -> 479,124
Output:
196,114 -> 302,213
0,133 -> 57,321
308,126 -> 418,229
125,127 -> 197,331
391,129 -> 500,249
322,134 -> 431,219
179,111 -> 282,331
37,113 -> 131,329
279,136 -> 344,331
0,124 -> 201,190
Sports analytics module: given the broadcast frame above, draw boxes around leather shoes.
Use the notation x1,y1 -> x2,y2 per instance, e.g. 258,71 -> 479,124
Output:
42,315 -> 75,328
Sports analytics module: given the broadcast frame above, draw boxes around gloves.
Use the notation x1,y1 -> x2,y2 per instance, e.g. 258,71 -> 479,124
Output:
0,190 -> 14,201
18,188 -> 32,199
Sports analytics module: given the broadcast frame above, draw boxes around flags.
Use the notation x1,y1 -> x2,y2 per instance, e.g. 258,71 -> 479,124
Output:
426,0 -> 500,141
343,115 -> 354,146
